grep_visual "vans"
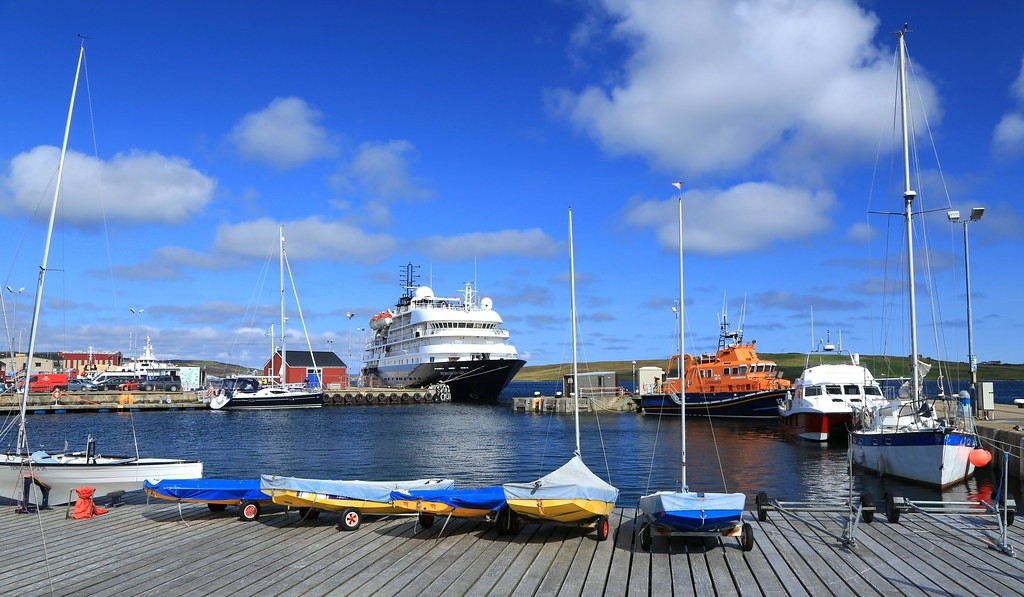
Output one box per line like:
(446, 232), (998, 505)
(16, 373), (68, 394)
(89, 371), (148, 390)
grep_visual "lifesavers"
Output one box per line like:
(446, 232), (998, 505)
(366, 393), (374, 406)
(53, 389), (63, 399)
(424, 393), (432, 404)
(333, 393), (342, 406)
(355, 393), (364, 406)
(413, 393), (421, 405)
(344, 393), (353, 406)
(401, 393), (409, 405)
(390, 392), (398, 406)
(321, 393), (331, 407)
(378, 393), (386, 406)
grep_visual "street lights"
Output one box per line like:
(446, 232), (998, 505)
(947, 207), (986, 417)
(129, 307), (144, 379)
(6, 286), (26, 395)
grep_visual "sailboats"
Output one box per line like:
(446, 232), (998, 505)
(846, 19), (985, 491)
(202, 223), (327, 409)
(639, 194), (746, 532)
(388, 200), (620, 519)
(0, 30), (212, 508)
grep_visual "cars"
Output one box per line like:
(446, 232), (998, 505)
(67, 378), (92, 392)
(98, 374), (182, 394)
(81, 378), (98, 392)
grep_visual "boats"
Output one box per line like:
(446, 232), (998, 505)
(260, 474), (456, 517)
(141, 478), (272, 507)
(775, 303), (890, 442)
(637, 288), (796, 422)
(357, 254), (527, 403)
